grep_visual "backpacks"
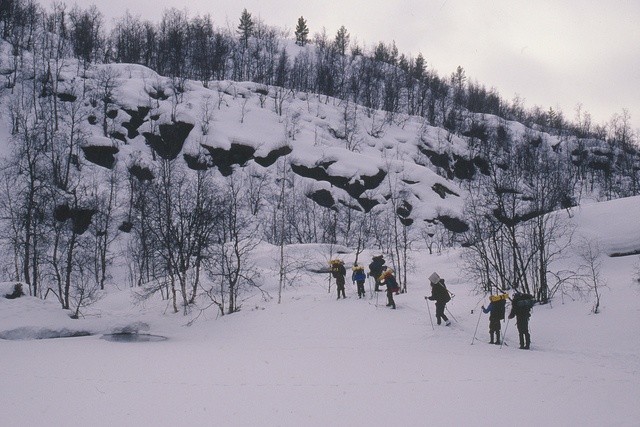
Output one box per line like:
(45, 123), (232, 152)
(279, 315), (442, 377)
(494, 299), (506, 320)
(332, 265), (342, 278)
(515, 299), (531, 311)
(368, 261), (384, 273)
(355, 271), (364, 280)
(435, 286), (450, 303)
(386, 274), (397, 292)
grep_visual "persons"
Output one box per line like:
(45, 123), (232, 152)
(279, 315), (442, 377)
(331, 260), (346, 298)
(481, 294), (509, 345)
(425, 272), (452, 326)
(377, 271), (400, 309)
(352, 265), (366, 298)
(369, 255), (387, 291)
(507, 292), (537, 349)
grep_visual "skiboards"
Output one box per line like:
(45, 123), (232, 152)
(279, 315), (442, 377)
(475, 338), (509, 345)
(368, 302), (404, 309)
